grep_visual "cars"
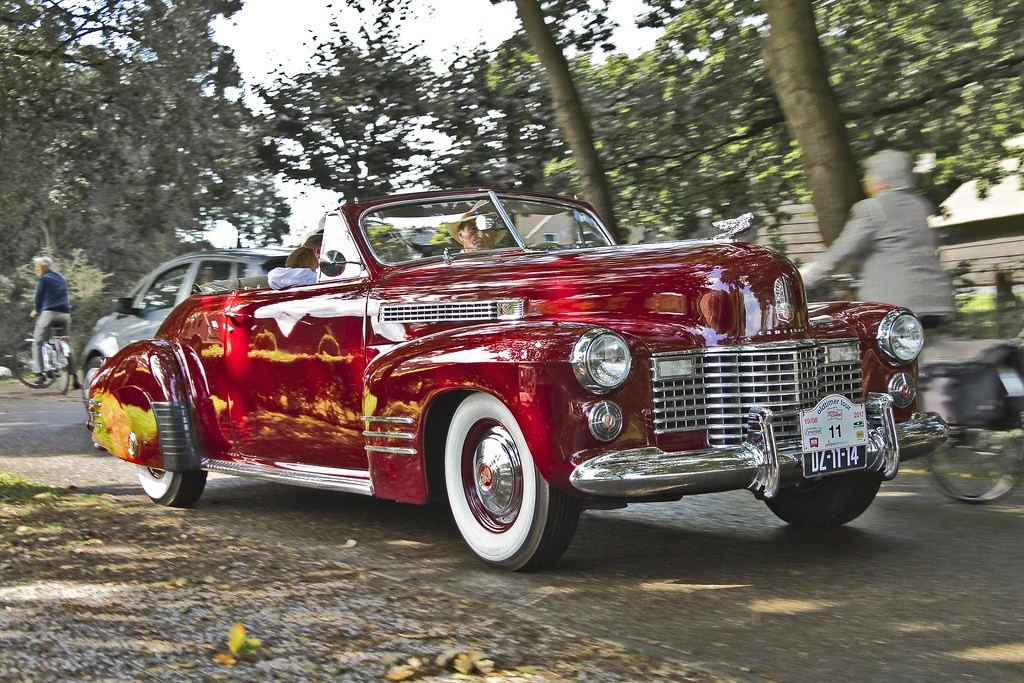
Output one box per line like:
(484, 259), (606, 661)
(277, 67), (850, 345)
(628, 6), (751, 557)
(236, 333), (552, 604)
(86, 187), (949, 576)
(79, 246), (302, 412)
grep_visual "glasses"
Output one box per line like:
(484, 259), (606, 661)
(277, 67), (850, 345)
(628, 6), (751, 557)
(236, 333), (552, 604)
(859, 174), (876, 184)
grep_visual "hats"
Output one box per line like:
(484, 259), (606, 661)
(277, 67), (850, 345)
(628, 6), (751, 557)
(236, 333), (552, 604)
(285, 214), (339, 268)
(444, 200), (508, 246)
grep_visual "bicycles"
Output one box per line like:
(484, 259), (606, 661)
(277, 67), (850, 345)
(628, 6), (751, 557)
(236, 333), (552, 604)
(827, 272), (1024, 504)
(11, 313), (73, 395)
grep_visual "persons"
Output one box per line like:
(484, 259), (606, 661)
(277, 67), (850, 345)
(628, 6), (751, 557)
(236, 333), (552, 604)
(796, 149), (956, 329)
(30, 257), (84, 391)
(268, 214), (325, 290)
(444, 206), (508, 257)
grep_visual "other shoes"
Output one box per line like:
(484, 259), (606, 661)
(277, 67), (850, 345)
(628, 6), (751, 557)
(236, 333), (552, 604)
(34, 375), (46, 384)
(73, 381), (83, 389)
